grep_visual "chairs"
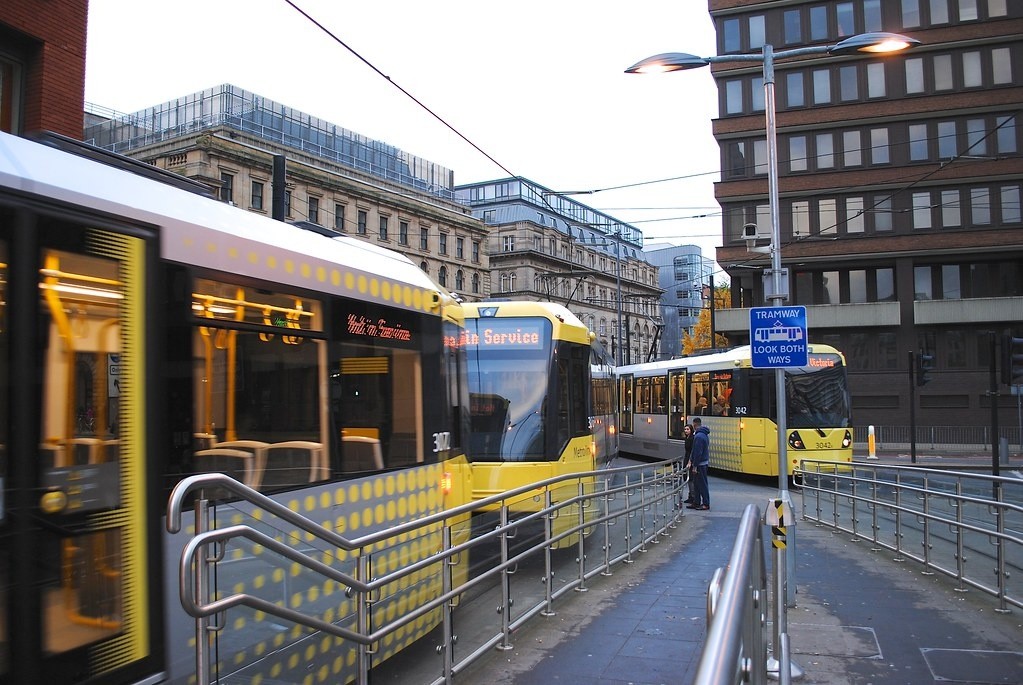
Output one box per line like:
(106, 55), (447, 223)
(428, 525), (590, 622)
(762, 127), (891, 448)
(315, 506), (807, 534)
(38, 431), (388, 629)
(631, 401), (729, 416)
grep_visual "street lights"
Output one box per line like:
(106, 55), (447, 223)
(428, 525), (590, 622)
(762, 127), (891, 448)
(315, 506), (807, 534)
(617, 32), (922, 611)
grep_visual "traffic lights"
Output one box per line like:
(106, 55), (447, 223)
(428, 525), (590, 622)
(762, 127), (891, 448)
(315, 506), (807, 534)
(916, 350), (933, 386)
(1000, 328), (1023, 387)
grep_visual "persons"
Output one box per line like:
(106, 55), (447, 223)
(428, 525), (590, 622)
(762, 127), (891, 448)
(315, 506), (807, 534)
(686, 418), (710, 511)
(656, 392), (731, 417)
(683, 424), (695, 504)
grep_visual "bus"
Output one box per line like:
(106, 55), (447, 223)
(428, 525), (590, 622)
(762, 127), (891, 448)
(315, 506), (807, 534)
(616, 342), (853, 487)
(460, 299), (621, 554)
(0, 127), (474, 685)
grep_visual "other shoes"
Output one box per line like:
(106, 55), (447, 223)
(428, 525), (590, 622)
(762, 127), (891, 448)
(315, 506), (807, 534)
(684, 499), (693, 503)
(695, 504), (709, 510)
(686, 503), (700, 509)
